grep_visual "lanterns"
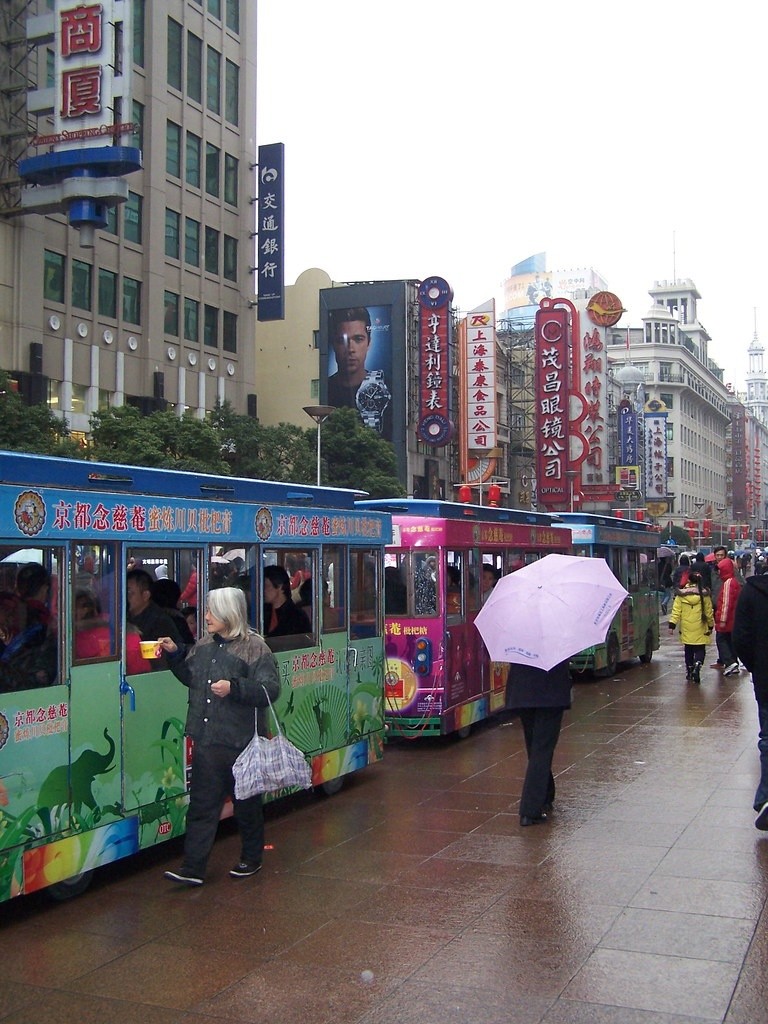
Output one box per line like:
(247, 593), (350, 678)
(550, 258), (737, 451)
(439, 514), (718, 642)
(488, 484), (501, 507)
(460, 486), (472, 504)
(615, 510), (762, 541)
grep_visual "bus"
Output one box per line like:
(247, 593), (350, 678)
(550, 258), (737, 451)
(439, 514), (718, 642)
(1, 451), (392, 909)
(548, 512), (661, 678)
(356, 498), (572, 747)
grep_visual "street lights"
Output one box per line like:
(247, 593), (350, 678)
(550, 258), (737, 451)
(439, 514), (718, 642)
(761, 518), (767, 547)
(736, 511), (742, 545)
(749, 515), (756, 543)
(717, 508), (726, 545)
(302, 406), (336, 486)
(663, 496), (676, 540)
(622, 486), (636, 521)
(694, 502), (704, 551)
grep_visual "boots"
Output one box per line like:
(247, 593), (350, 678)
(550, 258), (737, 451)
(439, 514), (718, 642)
(691, 661), (704, 682)
(686, 666), (694, 681)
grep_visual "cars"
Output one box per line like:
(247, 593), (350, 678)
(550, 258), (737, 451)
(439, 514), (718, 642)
(735, 539), (757, 548)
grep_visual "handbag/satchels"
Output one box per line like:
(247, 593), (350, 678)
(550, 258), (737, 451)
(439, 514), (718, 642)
(232, 684), (313, 799)
(658, 579), (665, 592)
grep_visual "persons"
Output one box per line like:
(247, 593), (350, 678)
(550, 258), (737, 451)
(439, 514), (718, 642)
(385, 566), (407, 615)
(297, 577), (339, 629)
(737, 555), (768, 575)
(504, 661), (575, 827)
(730, 555), (768, 831)
(157, 588), (281, 884)
(483, 563), (499, 607)
(290, 559), (313, 589)
(327, 306), (392, 441)
(127, 569), (184, 672)
(182, 607), (197, 643)
(426, 556), (461, 593)
(0, 562), (59, 694)
(177, 562), (197, 609)
(402, 555), (436, 615)
(73, 587), (153, 675)
(151, 579), (195, 645)
(263, 564), (312, 637)
(658, 546), (747, 683)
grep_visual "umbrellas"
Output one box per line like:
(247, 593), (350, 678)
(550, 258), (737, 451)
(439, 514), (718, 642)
(727, 550), (753, 555)
(657, 547), (675, 557)
(474, 553), (630, 672)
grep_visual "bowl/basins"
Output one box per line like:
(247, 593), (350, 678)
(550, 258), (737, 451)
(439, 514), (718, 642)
(139, 641), (163, 659)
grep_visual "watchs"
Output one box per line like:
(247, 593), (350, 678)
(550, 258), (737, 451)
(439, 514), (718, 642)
(356, 369), (391, 433)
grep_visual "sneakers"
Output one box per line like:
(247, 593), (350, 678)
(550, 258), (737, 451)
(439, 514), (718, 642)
(229, 862), (262, 877)
(163, 867), (204, 886)
(723, 663), (740, 676)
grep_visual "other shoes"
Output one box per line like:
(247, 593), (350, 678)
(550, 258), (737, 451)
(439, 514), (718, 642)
(710, 663), (726, 668)
(542, 802), (553, 813)
(755, 802), (768, 830)
(661, 603), (667, 615)
(521, 814), (547, 826)
